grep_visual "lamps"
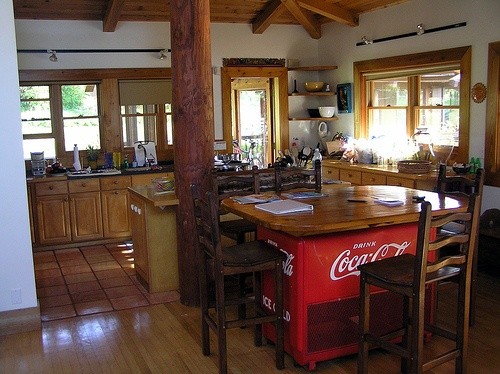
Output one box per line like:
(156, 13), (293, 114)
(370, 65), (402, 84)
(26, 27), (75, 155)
(160, 49), (168, 60)
(417, 24), (424, 35)
(361, 35), (373, 44)
(47, 49), (57, 62)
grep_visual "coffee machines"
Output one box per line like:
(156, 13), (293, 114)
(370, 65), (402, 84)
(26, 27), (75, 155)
(30, 152), (45, 175)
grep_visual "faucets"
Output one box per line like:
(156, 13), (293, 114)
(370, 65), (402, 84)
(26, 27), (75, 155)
(137, 144), (149, 167)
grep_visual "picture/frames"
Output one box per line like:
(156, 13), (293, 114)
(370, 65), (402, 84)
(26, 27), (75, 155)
(336, 83), (353, 113)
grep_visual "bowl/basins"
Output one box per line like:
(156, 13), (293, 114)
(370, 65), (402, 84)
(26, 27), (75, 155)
(317, 106), (337, 118)
(151, 176), (173, 192)
(304, 82), (325, 91)
(306, 109), (320, 118)
(452, 166), (471, 173)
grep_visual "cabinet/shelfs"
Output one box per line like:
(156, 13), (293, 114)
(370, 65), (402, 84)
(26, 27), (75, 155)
(362, 172), (386, 184)
(339, 168), (362, 184)
(322, 166), (340, 180)
(389, 176), (415, 189)
(288, 66), (338, 121)
(26, 172), (179, 293)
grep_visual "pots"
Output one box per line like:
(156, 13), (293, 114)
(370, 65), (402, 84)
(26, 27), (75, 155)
(230, 152), (241, 161)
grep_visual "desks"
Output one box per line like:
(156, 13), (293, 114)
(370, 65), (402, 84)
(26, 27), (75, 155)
(220, 185), (470, 374)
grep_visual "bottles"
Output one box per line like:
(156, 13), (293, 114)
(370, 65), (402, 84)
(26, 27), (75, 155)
(326, 84), (331, 92)
(291, 143), (299, 164)
(104, 149), (124, 167)
(312, 148), (322, 185)
(470, 157), (475, 173)
(474, 158), (481, 174)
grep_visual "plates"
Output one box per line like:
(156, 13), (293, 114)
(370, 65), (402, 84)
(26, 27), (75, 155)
(46, 173), (67, 176)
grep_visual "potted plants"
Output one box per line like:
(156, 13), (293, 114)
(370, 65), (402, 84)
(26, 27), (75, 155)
(86, 145), (100, 169)
(470, 158), (481, 179)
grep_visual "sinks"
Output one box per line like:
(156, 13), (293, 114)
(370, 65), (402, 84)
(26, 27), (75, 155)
(66, 170), (88, 177)
(89, 169), (122, 176)
(125, 167), (151, 172)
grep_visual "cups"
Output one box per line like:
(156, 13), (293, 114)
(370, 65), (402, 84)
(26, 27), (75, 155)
(223, 153), (230, 162)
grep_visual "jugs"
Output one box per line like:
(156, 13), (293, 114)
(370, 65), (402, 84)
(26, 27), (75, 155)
(31, 160), (44, 174)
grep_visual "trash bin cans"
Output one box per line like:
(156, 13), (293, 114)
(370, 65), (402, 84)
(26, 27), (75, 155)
(477, 208), (500, 278)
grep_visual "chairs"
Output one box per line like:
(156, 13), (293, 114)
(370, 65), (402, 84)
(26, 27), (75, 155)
(189, 159), (485, 374)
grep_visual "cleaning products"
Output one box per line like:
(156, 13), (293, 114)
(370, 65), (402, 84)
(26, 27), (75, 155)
(124, 154), (138, 168)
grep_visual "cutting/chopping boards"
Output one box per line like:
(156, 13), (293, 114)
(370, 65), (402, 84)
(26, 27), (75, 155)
(289, 137), (306, 150)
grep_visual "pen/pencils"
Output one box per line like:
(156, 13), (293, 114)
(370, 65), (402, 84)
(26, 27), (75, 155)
(347, 200), (367, 202)
